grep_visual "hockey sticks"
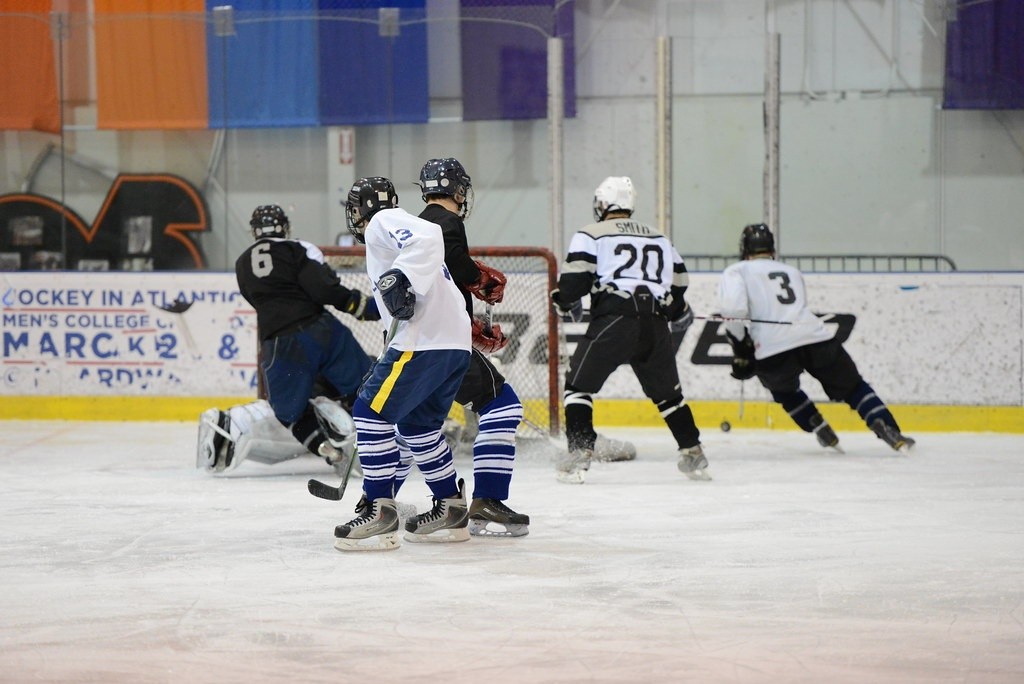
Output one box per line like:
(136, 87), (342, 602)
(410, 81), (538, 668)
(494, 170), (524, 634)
(739, 379), (745, 423)
(307, 317), (400, 501)
(484, 300), (492, 337)
(581, 308), (792, 325)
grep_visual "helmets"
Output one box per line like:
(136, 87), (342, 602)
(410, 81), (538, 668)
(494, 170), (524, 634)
(592, 176), (635, 223)
(739, 222), (774, 260)
(419, 158), (474, 218)
(345, 176), (398, 244)
(250, 205), (289, 240)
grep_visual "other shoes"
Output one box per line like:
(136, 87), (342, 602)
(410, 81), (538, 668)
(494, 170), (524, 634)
(196, 407), (229, 468)
(309, 396), (355, 447)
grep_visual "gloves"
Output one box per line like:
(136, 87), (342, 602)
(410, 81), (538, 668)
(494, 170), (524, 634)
(374, 268), (416, 320)
(726, 326), (756, 380)
(472, 321), (506, 353)
(662, 302), (693, 333)
(465, 260), (507, 306)
(549, 289), (582, 323)
(349, 289), (381, 321)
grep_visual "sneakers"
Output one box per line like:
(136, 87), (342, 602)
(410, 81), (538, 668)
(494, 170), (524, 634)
(334, 482), (401, 551)
(677, 444), (712, 481)
(467, 497), (529, 537)
(555, 448), (593, 484)
(355, 493), (417, 531)
(810, 413), (845, 453)
(868, 418), (915, 457)
(403, 478), (471, 543)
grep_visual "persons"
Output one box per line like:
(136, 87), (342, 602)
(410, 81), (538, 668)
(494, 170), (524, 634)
(202, 395), (461, 476)
(334, 176), (474, 540)
(235, 205), (381, 477)
(721, 223), (916, 455)
(355, 158), (529, 525)
(550, 176), (709, 473)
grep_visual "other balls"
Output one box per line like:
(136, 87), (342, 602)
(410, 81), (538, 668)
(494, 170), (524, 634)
(721, 421), (730, 432)
